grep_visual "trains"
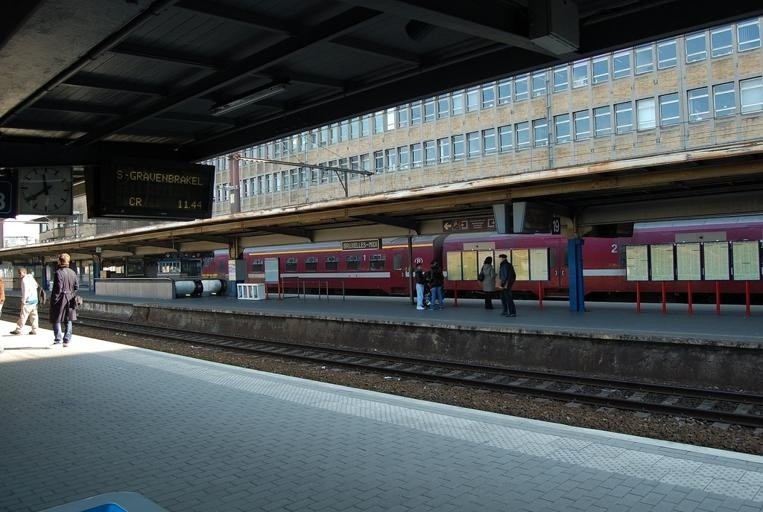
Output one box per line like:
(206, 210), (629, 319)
(199, 215), (763, 298)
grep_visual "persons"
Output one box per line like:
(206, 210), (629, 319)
(415, 263), (425, 309)
(10, 268), (38, 335)
(482, 257), (498, 309)
(49, 253), (79, 347)
(499, 255), (516, 317)
(426, 260), (444, 310)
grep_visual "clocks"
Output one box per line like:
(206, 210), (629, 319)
(20, 165), (73, 217)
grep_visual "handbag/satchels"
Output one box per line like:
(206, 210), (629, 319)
(72, 295), (83, 308)
(478, 273), (484, 281)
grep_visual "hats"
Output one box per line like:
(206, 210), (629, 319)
(499, 254), (506, 257)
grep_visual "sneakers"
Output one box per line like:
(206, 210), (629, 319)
(505, 314), (515, 317)
(29, 332), (35, 334)
(10, 331), (18, 334)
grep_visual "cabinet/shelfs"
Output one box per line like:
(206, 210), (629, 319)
(236, 282), (267, 301)
